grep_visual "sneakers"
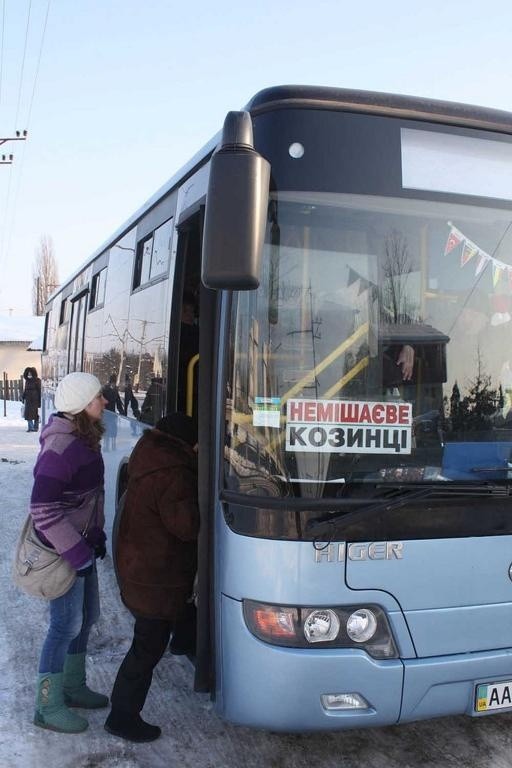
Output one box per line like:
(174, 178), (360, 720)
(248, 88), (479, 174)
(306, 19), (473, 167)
(102, 708), (161, 741)
(170, 632), (196, 654)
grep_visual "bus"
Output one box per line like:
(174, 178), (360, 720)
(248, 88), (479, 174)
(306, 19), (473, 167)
(36, 82), (512, 735)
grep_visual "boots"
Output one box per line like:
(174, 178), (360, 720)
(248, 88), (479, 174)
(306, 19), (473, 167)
(33, 672), (89, 733)
(62, 652), (108, 707)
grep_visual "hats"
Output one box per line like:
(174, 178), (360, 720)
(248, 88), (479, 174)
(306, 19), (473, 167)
(55, 373), (102, 415)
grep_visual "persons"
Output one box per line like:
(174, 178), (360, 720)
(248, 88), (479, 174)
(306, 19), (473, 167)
(20, 367), (41, 432)
(104, 413), (199, 742)
(180, 289), (198, 375)
(30, 370), (109, 734)
(396, 343), (417, 381)
(101, 374), (166, 451)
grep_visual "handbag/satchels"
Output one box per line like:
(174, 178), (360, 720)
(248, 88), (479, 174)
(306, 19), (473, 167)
(12, 514), (88, 600)
